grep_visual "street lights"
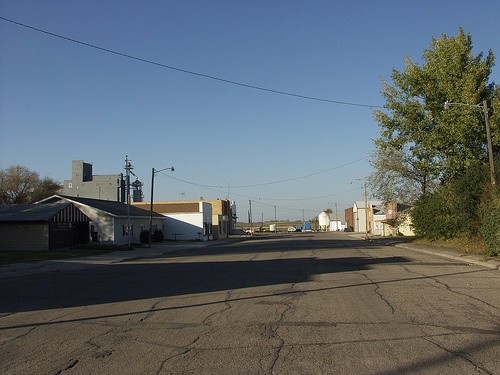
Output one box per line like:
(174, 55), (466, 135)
(444, 98), (494, 184)
(349, 181), (368, 239)
(148, 167), (176, 248)
